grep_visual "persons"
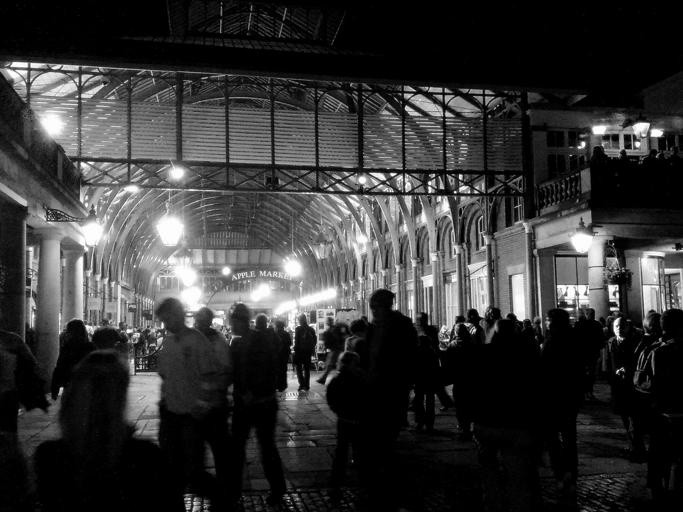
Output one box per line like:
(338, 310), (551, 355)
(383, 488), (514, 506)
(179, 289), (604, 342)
(584, 144), (683, 168)
(0, 288), (683, 512)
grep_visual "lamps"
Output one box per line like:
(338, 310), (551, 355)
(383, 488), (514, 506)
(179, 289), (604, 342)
(311, 194), (333, 260)
(620, 113), (650, 137)
(43, 205), (102, 247)
(156, 180), (202, 309)
